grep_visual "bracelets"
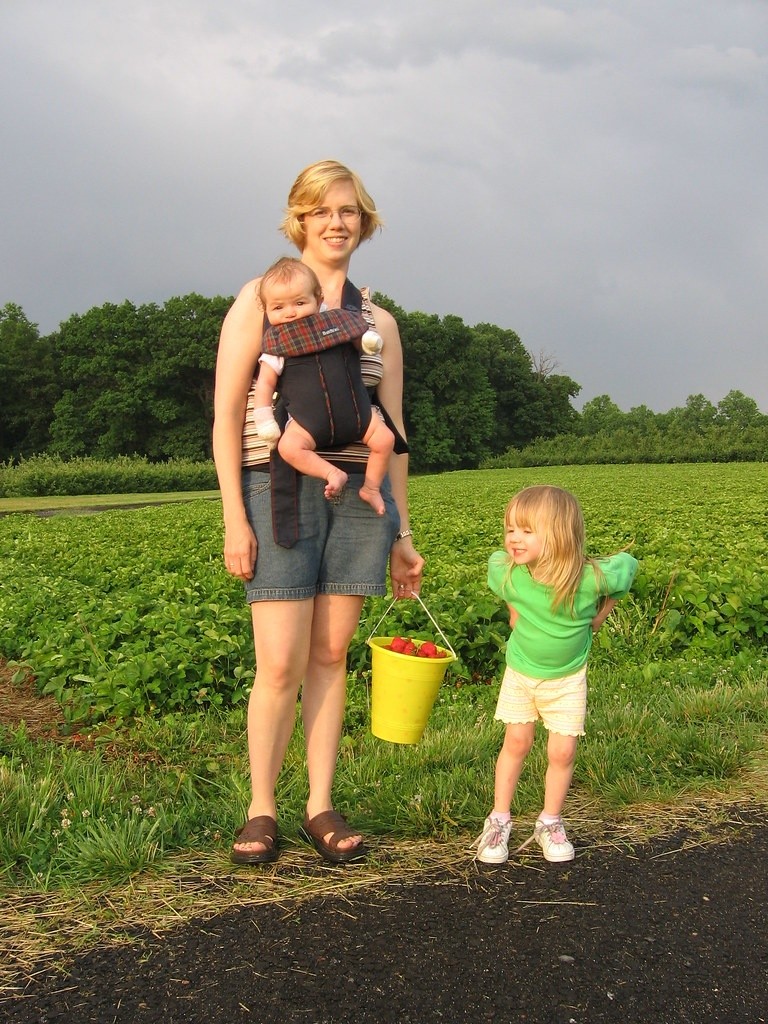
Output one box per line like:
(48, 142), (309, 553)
(396, 530), (413, 542)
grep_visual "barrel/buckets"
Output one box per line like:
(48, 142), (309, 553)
(364, 590), (457, 746)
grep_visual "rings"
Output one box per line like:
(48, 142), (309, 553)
(230, 564), (234, 566)
(399, 585), (404, 588)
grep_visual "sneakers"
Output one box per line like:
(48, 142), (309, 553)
(530, 819), (574, 863)
(468, 812), (513, 866)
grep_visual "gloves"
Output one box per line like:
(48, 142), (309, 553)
(363, 330), (384, 356)
(251, 406), (282, 449)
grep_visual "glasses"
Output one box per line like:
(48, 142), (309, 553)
(308, 205), (362, 224)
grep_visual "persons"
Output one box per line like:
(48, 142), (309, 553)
(474, 486), (637, 863)
(210, 161), (424, 864)
(251, 257), (395, 514)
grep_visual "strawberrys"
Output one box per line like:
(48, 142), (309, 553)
(379, 635), (447, 658)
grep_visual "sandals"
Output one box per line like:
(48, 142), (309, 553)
(298, 805), (366, 863)
(232, 815), (280, 864)
